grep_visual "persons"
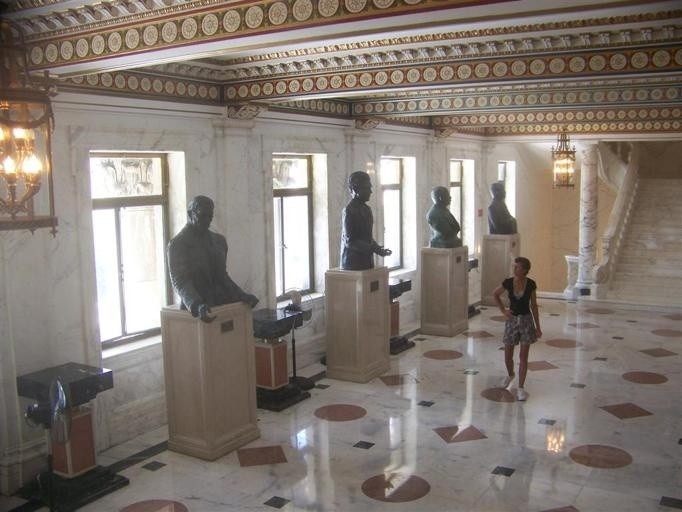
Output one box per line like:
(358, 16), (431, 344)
(491, 256), (542, 401)
(486, 182), (517, 234)
(425, 185), (462, 247)
(340, 170), (392, 270)
(167, 194), (259, 323)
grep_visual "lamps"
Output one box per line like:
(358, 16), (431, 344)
(0, 15), (61, 239)
(550, 122), (576, 190)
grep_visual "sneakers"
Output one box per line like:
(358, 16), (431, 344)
(517, 388), (526, 400)
(501, 372), (516, 389)
